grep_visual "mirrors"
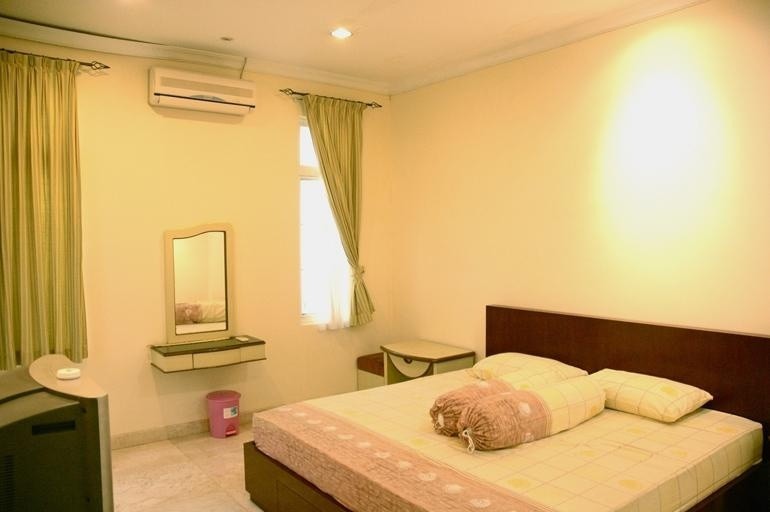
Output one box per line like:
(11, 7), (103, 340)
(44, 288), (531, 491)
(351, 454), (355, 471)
(165, 223), (232, 342)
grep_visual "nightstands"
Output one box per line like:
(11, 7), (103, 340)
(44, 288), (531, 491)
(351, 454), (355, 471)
(380, 339), (475, 385)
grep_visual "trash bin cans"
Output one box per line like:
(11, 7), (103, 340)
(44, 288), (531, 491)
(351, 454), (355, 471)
(205, 390), (241, 438)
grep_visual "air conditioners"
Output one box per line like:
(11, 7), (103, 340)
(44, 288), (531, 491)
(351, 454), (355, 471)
(146, 65), (256, 115)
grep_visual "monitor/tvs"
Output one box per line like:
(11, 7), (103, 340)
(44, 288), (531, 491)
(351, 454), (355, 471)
(0, 353), (114, 512)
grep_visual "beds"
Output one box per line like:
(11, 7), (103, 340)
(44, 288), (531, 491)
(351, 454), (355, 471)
(244, 304), (769, 512)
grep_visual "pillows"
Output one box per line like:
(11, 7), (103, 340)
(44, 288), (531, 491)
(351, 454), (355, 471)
(429, 352), (713, 454)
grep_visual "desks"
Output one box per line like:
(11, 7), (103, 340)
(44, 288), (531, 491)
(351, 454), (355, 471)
(149, 334), (266, 374)
